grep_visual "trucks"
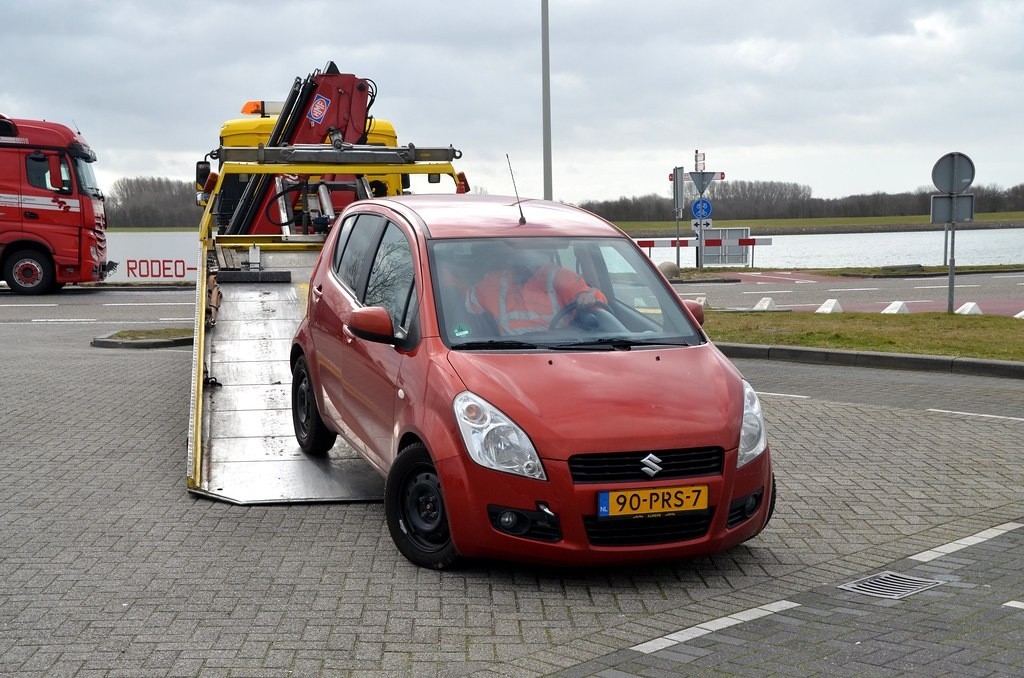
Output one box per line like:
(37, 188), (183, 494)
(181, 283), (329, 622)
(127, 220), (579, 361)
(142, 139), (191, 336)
(0, 112), (119, 294)
(185, 57), (476, 506)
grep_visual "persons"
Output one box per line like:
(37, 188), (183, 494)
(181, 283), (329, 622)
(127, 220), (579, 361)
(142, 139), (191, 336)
(440, 242), (607, 338)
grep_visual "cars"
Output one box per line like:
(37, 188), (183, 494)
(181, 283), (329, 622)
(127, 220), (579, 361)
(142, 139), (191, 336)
(288, 193), (778, 572)
